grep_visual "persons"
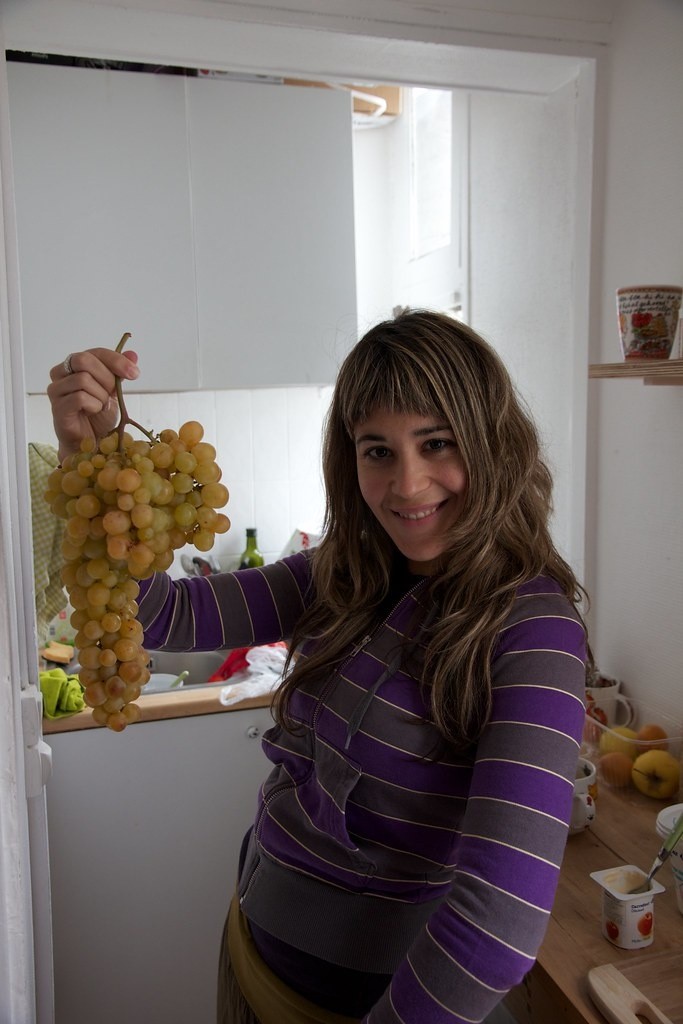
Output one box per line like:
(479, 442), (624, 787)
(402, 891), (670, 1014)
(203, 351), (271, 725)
(47, 312), (594, 1024)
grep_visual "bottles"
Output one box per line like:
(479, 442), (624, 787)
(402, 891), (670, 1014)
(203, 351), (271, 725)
(238, 528), (264, 571)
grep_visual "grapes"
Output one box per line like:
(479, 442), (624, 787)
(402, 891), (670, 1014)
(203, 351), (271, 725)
(43, 333), (233, 733)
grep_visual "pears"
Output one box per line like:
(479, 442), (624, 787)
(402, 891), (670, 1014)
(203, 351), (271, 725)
(631, 751), (681, 797)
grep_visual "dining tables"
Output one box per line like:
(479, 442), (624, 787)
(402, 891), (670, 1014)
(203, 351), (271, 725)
(503, 741), (683, 1024)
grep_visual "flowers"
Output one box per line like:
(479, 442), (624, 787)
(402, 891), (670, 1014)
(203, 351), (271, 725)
(630, 312), (657, 353)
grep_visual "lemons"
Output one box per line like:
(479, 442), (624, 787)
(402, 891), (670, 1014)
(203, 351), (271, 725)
(600, 727), (637, 757)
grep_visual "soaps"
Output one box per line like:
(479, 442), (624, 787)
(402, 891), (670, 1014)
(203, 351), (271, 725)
(42, 638), (75, 665)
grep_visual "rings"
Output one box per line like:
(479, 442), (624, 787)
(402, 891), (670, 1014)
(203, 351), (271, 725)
(64, 353), (75, 374)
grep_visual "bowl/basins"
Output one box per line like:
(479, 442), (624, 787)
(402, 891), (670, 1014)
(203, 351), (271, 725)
(139, 673), (184, 692)
(581, 696), (683, 808)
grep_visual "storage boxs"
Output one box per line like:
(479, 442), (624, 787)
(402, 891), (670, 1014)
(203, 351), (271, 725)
(579, 696), (683, 805)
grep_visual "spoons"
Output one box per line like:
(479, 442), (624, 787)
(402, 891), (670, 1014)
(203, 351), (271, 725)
(625, 810), (683, 896)
(180, 553), (222, 578)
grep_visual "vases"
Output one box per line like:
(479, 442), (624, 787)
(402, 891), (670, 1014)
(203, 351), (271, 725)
(618, 285), (683, 363)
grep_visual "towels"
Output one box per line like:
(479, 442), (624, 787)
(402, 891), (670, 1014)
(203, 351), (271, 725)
(36, 667), (94, 719)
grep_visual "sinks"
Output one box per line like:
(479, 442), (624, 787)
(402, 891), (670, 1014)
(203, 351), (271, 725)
(46, 647), (253, 697)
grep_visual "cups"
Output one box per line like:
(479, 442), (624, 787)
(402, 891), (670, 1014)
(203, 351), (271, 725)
(614, 284), (683, 362)
(583, 671), (636, 740)
(566, 756), (597, 835)
(654, 802), (682, 917)
